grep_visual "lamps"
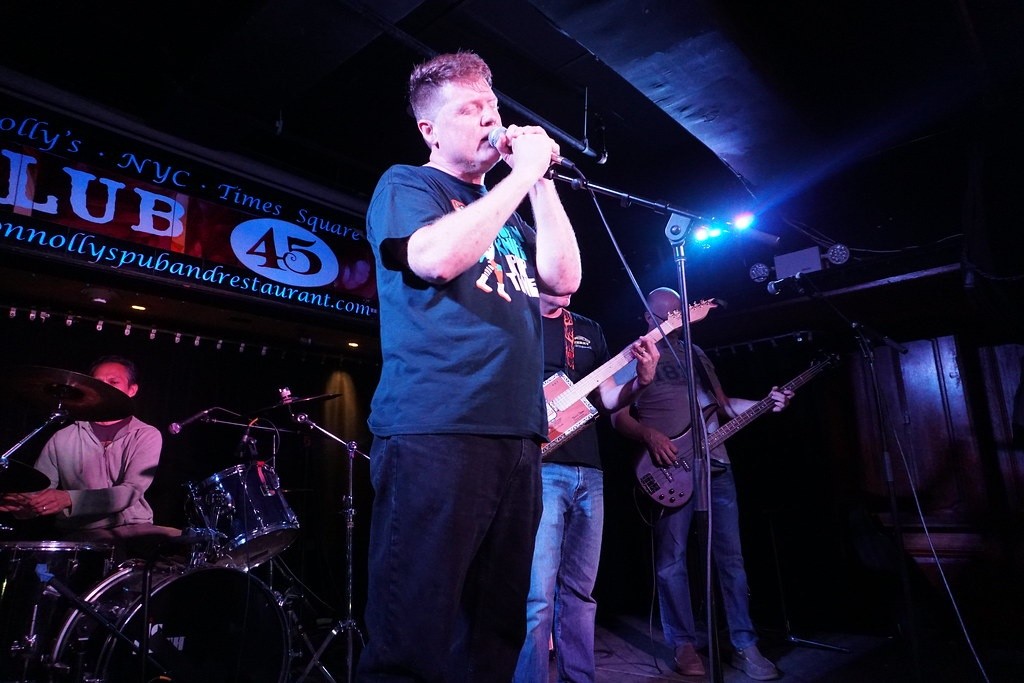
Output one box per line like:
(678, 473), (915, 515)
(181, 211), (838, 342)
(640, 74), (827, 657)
(749, 244), (850, 283)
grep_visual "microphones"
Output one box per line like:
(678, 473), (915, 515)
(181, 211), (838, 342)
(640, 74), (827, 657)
(488, 126), (575, 168)
(169, 408), (213, 434)
(237, 428), (252, 458)
(768, 272), (803, 294)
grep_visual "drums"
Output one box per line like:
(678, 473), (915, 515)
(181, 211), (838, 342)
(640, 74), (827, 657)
(48, 559), (292, 683)
(0, 540), (120, 683)
(183, 462), (301, 573)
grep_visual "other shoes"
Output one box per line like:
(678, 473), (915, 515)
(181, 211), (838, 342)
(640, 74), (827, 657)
(732, 645), (778, 679)
(674, 643), (704, 675)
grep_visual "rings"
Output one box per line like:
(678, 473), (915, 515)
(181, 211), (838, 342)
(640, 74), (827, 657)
(44, 507), (46, 509)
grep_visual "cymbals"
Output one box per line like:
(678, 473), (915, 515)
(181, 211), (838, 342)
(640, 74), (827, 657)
(0, 364), (136, 421)
(240, 392), (344, 418)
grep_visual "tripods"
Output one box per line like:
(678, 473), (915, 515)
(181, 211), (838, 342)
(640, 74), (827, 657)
(286, 402), (373, 683)
(754, 504), (854, 661)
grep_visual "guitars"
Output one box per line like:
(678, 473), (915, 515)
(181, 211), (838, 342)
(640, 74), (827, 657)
(635, 348), (843, 511)
(540, 296), (717, 460)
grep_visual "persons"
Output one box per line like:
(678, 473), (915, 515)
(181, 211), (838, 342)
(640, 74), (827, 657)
(365, 54), (585, 682)
(1, 358), (162, 537)
(515, 293), (660, 680)
(609, 289), (798, 679)
